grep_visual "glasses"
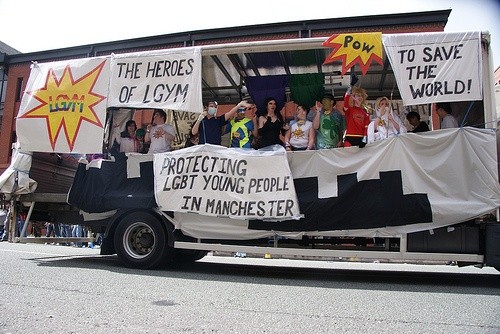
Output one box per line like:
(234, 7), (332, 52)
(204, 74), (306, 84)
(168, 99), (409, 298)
(154, 114), (160, 118)
(244, 107), (254, 111)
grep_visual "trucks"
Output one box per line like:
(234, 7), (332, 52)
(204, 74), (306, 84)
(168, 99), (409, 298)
(1, 28), (500, 270)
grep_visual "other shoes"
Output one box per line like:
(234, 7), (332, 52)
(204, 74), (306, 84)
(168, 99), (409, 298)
(0, 238), (8, 242)
(45, 241), (94, 249)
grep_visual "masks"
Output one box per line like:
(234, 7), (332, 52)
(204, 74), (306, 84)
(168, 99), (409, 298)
(207, 108), (217, 115)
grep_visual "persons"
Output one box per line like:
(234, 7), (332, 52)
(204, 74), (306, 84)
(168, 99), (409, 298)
(103, 87), (464, 155)
(0, 196), (104, 248)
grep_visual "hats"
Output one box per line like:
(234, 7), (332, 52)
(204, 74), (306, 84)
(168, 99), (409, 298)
(320, 93), (336, 106)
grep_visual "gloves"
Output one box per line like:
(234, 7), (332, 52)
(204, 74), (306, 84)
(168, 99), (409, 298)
(197, 113), (205, 121)
(237, 100), (249, 108)
